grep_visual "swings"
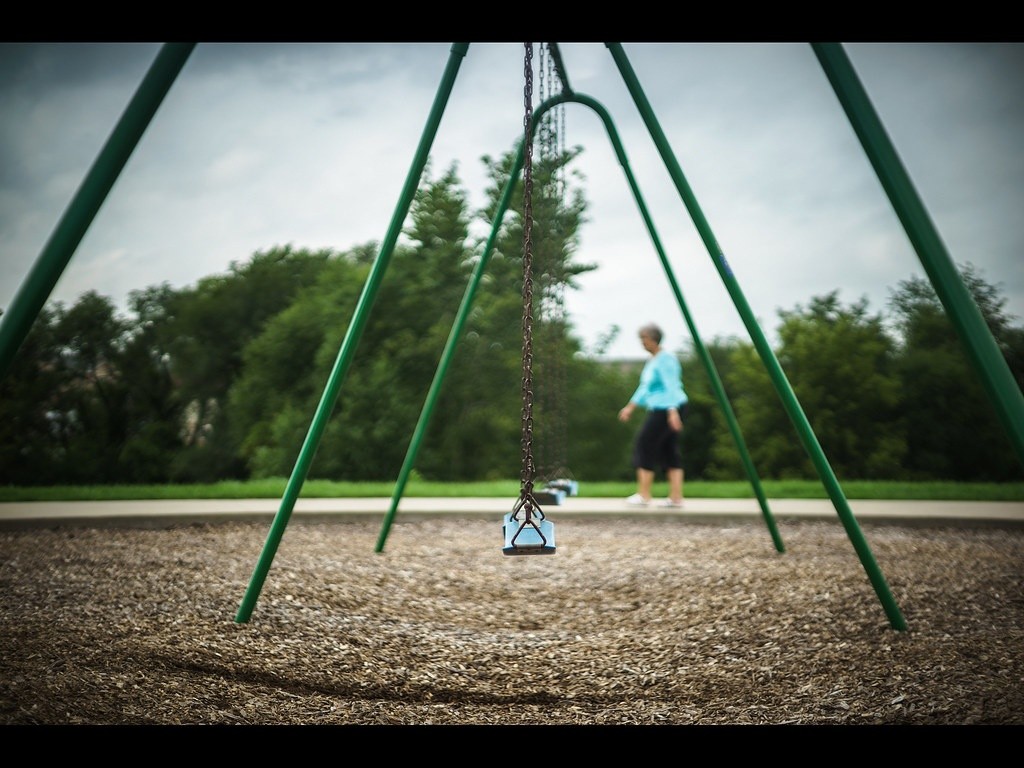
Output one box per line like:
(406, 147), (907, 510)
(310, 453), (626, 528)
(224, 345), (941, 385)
(501, 43), (586, 560)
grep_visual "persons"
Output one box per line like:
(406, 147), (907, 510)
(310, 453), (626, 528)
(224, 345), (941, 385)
(618, 324), (688, 508)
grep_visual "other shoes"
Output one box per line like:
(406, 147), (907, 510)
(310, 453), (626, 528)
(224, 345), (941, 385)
(660, 497), (683, 509)
(625, 493), (652, 508)
